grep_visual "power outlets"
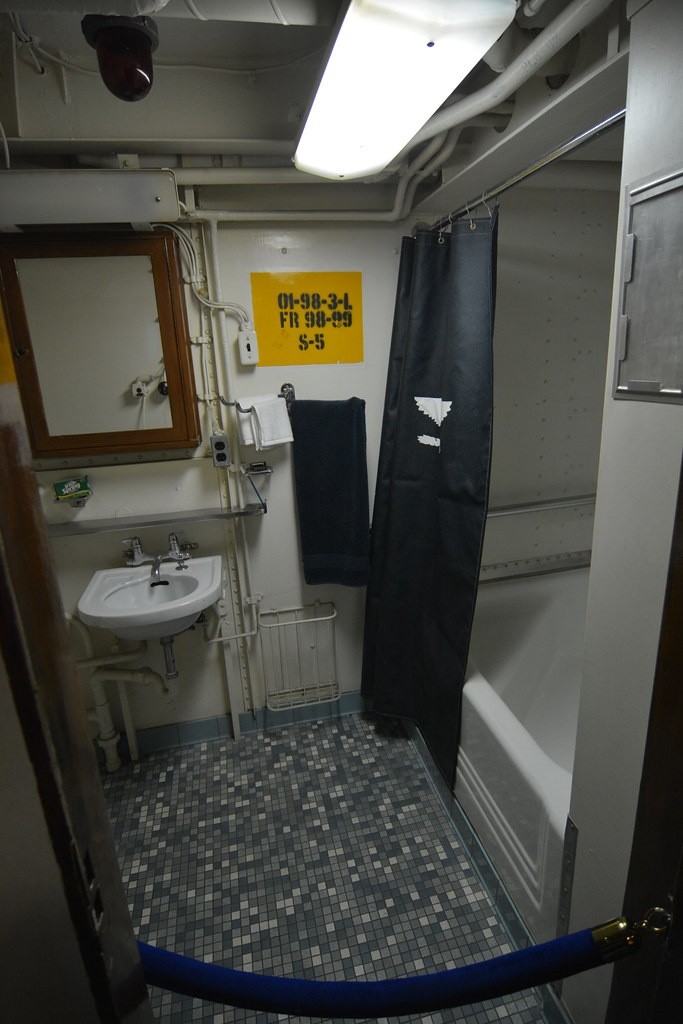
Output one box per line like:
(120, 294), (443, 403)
(211, 434), (232, 468)
(131, 382), (146, 397)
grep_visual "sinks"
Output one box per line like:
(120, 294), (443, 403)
(76, 554), (223, 641)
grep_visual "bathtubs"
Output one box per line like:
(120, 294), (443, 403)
(457, 564), (594, 872)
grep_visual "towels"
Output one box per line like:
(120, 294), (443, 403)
(234, 393), (295, 453)
(288, 396), (372, 589)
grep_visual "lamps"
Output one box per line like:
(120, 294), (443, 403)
(291, 0), (521, 180)
(81, 13), (159, 101)
(0, 167), (180, 226)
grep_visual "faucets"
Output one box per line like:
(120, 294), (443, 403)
(148, 551), (162, 583)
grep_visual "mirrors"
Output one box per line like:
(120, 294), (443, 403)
(0, 237), (190, 451)
